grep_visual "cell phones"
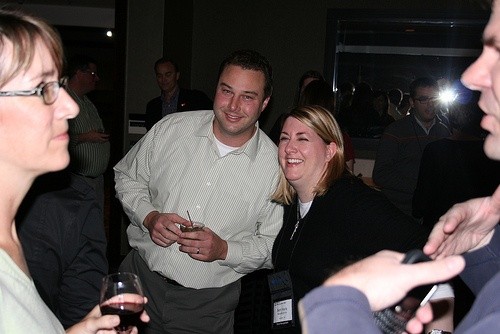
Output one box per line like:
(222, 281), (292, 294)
(371, 249), (438, 334)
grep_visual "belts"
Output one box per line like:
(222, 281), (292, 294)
(153, 271), (184, 287)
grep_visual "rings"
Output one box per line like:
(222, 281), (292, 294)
(196, 248), (200, 254)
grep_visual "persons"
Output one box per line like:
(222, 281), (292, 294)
(266, 105), (429, 334)
(0, 10), (151, 334)
(297, 0), (499, 334)
(270, 71), (355, 175)
(113, 50), (284, 334)
(339, 80), (452, 134)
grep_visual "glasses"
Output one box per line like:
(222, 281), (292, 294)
(0, 76), (71, 105)
(415, 96), (439, 104)
(85, 70), (95, 76)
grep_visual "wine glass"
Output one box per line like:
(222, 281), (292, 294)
(100, 271), (144, 334)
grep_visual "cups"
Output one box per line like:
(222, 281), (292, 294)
(96, 127), (108, 144)
(179, 221), (205, 249)
